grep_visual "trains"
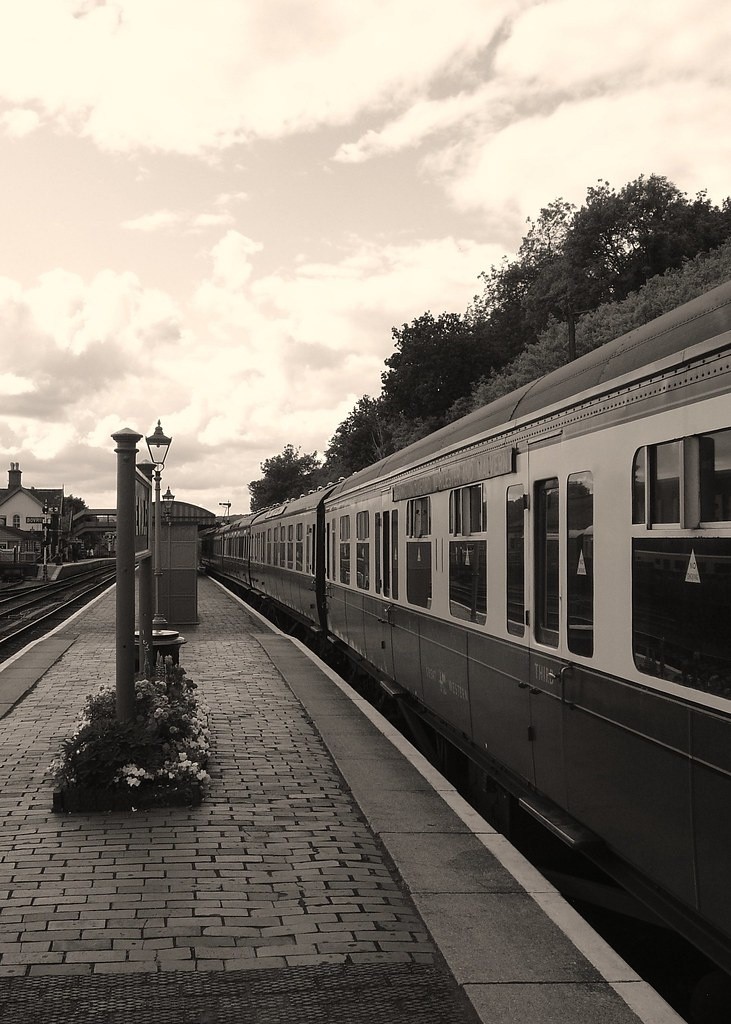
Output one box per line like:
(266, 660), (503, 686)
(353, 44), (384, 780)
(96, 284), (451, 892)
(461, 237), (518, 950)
(199, 281), (731, 975)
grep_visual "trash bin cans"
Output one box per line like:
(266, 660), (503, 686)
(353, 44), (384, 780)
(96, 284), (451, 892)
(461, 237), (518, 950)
(135, 630), (187, 672)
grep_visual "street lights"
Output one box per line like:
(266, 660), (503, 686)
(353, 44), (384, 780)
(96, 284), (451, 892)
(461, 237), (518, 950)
(162, 486), (175, 568)
(145, 419), (172, 612)
